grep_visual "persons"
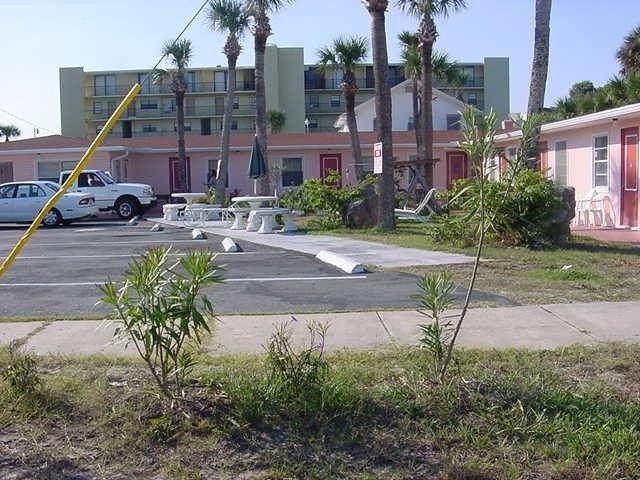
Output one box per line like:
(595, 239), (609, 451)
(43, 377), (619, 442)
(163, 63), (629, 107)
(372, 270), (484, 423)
(206, 172), (213, 189)
(206, 169), (216, 197)
(87, 174), (101, 186)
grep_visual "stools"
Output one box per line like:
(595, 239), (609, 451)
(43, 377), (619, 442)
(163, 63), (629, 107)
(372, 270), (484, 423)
(228, 207), (299, 234)
(162, 203), (221, 222)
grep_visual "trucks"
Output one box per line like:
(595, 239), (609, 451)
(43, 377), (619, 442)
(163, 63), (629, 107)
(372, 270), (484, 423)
(59, 169), (158, 220)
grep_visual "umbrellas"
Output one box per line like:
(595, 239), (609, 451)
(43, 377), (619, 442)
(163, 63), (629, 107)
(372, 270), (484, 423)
(247, 135), (267, 195)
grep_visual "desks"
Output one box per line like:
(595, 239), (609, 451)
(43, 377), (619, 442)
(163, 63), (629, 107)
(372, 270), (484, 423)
(231, 196), (280, 232)
(171, 192), (206, 221)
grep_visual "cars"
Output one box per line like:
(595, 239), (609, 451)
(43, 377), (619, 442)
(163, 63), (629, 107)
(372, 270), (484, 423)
(0, 180), (98, 229)
